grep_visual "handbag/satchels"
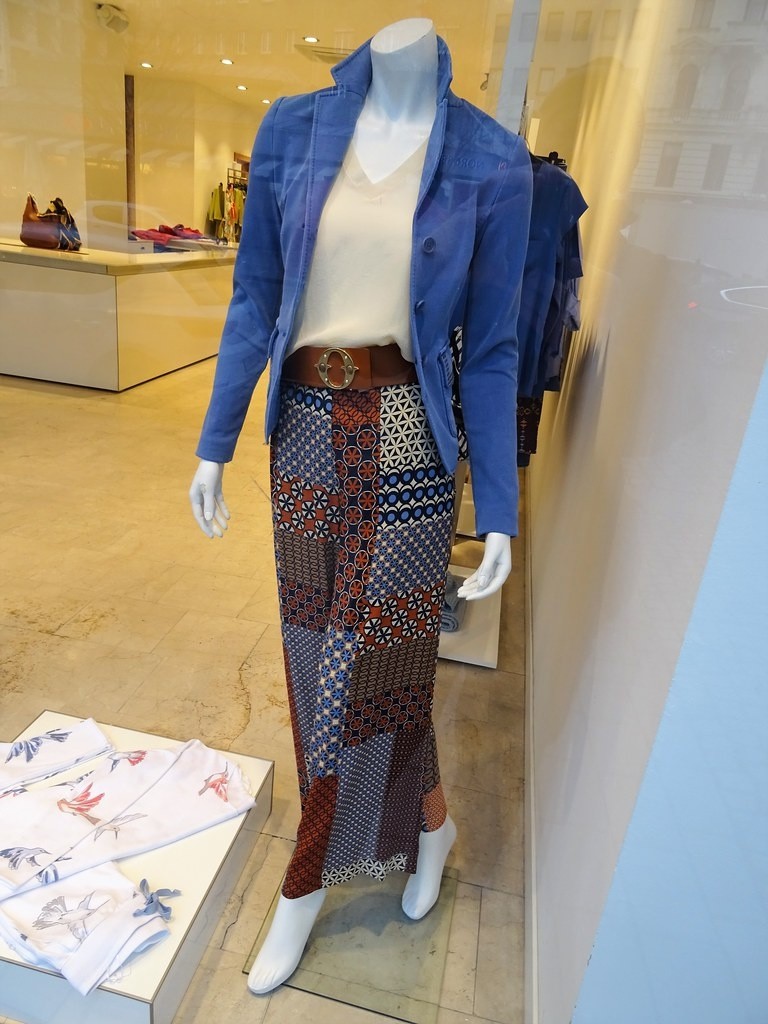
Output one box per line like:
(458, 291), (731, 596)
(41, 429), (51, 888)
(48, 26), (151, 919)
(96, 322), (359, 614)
(20, 192), (82, 252)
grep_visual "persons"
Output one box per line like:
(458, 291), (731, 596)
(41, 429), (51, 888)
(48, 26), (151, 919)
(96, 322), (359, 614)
(189, 16), (535, 995)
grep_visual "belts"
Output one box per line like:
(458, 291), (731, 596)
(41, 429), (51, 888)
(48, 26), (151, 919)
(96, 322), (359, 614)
(280, 344), (419, 389)
